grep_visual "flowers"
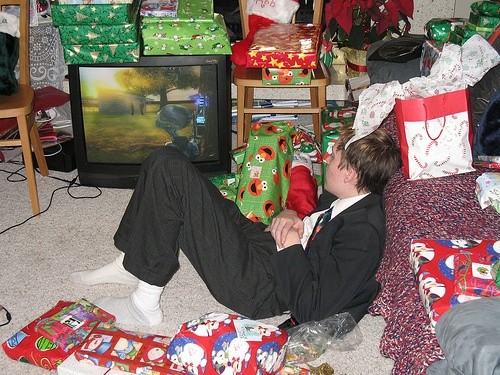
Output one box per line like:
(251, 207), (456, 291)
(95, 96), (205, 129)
(325, 0), (415, 51)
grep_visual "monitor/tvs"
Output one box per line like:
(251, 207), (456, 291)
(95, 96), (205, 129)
(68, 55), (233, 188)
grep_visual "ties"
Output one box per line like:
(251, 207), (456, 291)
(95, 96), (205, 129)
(305, 206), (339, 250)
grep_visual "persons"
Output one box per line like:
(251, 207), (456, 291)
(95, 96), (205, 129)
(70, 124), (402, 326)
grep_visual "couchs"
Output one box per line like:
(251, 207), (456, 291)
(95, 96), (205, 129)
(367, 112), (500, 375)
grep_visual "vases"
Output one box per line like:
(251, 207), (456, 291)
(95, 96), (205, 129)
(344, 45), (367, 77)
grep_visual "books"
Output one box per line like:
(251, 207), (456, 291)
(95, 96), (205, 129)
(251, 114), (299, 123)
(253, 100), (299, 108)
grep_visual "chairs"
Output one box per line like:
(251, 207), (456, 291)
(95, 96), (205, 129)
(234, 0), (340, 183)
(0, 0), (49, 217)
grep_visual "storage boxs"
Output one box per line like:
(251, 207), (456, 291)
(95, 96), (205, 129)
(424, 0), (500, 47)
(50, 0), (232, 64)
(2, 299), (289, 375)
(408, 239), (500, 334)
(246, 23), (322, 84)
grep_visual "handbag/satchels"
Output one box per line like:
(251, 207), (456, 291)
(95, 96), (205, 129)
(394, 84), (477, 182)
